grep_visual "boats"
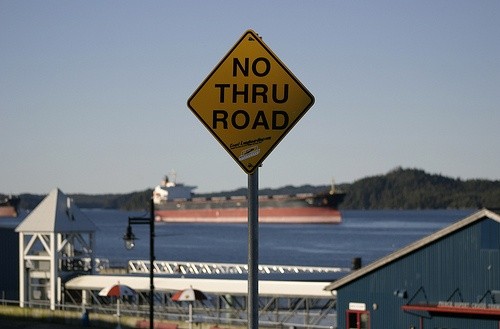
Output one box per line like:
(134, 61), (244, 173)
(151, 175), (347, 225)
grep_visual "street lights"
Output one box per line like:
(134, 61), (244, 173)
(123, 197), (155, 328)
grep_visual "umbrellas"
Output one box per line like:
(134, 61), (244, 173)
(170, 284), (210, 329)
(97, 280), (138, 329)
